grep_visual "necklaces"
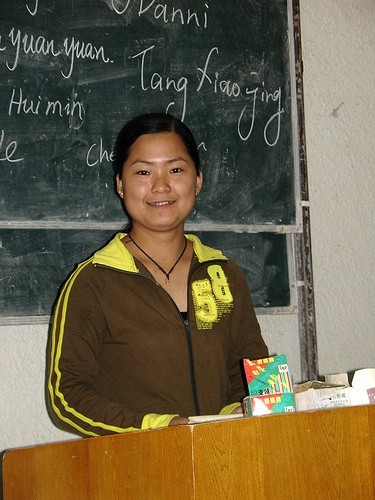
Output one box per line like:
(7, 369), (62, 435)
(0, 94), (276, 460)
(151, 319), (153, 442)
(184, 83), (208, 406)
(128, 232), (188, 285)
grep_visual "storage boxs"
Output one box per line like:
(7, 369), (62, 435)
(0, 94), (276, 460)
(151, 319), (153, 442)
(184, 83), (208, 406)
(243, 357), (375, 416)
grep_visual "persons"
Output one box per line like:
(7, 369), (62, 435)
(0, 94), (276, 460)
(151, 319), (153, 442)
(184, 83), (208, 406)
(44, 111), (269, 438)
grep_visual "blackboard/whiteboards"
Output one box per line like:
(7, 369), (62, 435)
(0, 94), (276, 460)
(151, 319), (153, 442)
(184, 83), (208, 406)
(0, 1), (304, 324)
